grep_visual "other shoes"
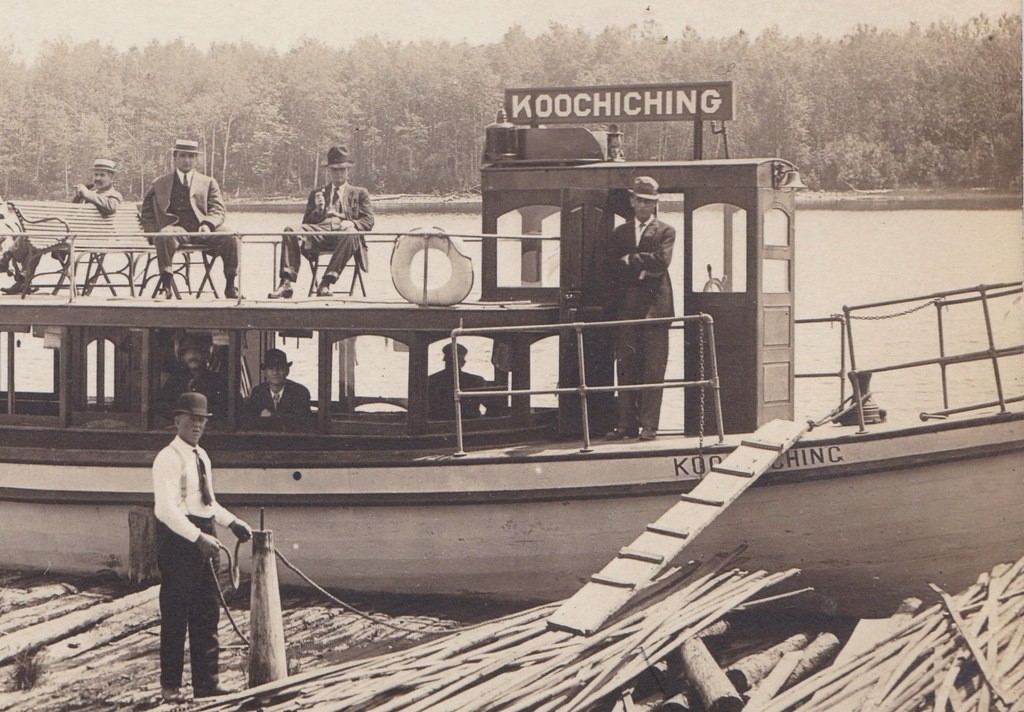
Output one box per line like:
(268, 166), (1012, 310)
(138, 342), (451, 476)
(0, 281), (26, 293)
(0, 258), (9, 272)
(161, 689), (193, 703)
(640, 426), (657, 440)
(193, 684), (241, 698)
(154, 288), (172, 301)
(316, 283), (333, 297)
(605, 426), (638, 440)
(269, 280), (293, 298)
(225, 287), (246, 299)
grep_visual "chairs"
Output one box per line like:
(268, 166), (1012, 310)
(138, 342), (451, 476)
(295, 243), (372, 297)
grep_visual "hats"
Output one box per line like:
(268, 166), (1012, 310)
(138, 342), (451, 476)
(261, 349), (293, 369)
(627, 176), (661, 200)
(171, 139), (203, 153)
(176, 334), (212, 357)
(91, 159), (120, 173)
(174, 393), (212, 416)
(443, 342), (467, 365)
(322, 147), (353, 168)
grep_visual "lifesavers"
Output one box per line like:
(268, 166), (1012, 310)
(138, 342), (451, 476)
(390, 226), (474, 306)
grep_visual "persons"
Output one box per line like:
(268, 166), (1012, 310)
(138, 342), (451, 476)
(141, 138), (247, 299)
(605, 177), (675, 438)
(239, 348), (310, 432)
(268, 145), (375, 299)
(0, 157), (123, 295)
(152, 392), (253, 702)
(429, 343), (494, 420)
(160, 336), (227, 412)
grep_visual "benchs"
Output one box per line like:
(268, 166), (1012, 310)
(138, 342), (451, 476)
(0, 201), (226, 303)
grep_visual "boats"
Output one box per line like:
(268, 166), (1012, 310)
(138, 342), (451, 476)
(1, 74), (1024, 648)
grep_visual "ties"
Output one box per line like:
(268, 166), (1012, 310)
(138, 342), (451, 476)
(273, 394), (279, 411)
(183, 175), (187, 186)
(639, 223), (645, 231)
(333, 187), (343, 214)
(193, 448), (213, 506)
